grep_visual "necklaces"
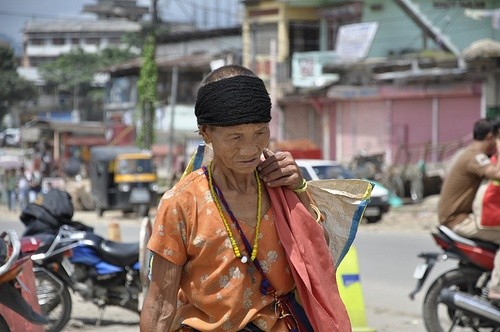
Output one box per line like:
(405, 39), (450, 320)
(207, 158), (263, 267)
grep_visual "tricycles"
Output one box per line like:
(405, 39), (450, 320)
(89, 145), (156, 219)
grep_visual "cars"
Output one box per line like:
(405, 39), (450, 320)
(295, 158), (390, 224)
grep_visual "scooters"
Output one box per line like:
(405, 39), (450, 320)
(0, 224), (96, 332)
(17, 182), (141, 328)
(409, 225), (500, 332)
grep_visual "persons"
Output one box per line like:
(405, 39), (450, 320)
(140, 65), (330, 332)
(438, 118), (500, 300)
(0, 149), (51, 213)
